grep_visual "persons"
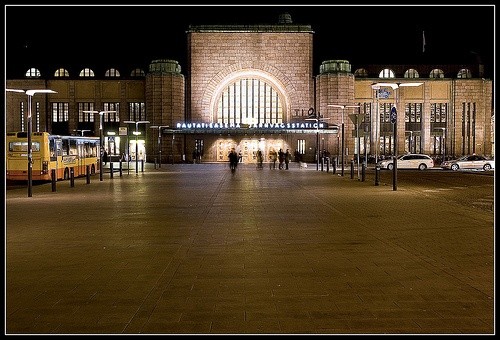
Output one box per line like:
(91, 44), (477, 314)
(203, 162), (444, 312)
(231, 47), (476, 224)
(268, 147), (278, 169)
(284, 149), (293, 170)
(119, 151), (128, 162)
(227, 148), (239, 181)
(278, 148), (285, 171)
(256, 148), (263, 170)
(238, 150), (243, 162)
(192, 149), (197, 164)
(103, 150), (107, 166)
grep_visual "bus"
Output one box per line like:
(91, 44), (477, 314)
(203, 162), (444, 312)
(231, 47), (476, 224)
(6, 132), (99, 182)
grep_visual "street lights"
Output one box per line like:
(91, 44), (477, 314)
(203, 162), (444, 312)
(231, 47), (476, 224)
(328, 105), (362, 176)
(73, 130), (91, 136)
(370, 82), (423, 190)
(305, 118), (330, 171)
(149, 125), (169, 164)
(82, 111), (116, 181)
(123, 121), (150, 173)
(6, 89), (58, 197)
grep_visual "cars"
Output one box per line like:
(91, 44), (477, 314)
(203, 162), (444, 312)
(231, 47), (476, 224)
(440, 155), (495, 171)
(378, 154), (434, 170)
